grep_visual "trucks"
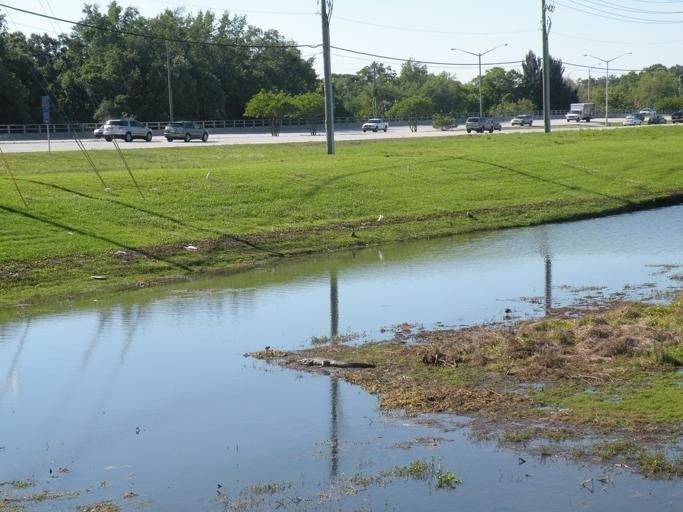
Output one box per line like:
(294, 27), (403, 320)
(565, 102), (596, 122)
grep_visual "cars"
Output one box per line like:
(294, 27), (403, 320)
(465, 116), (502, 133)
(164, 119), (210, 142)
(361, 118), (388, 132)
(623, 107), (683, 126)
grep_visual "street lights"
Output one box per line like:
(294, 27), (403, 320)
(450, 43), (508, 117)
(583, 51), (632, 125)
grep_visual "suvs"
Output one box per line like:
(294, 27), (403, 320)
(93, 119), (153, 142)
(510, 114), (532, 126)
